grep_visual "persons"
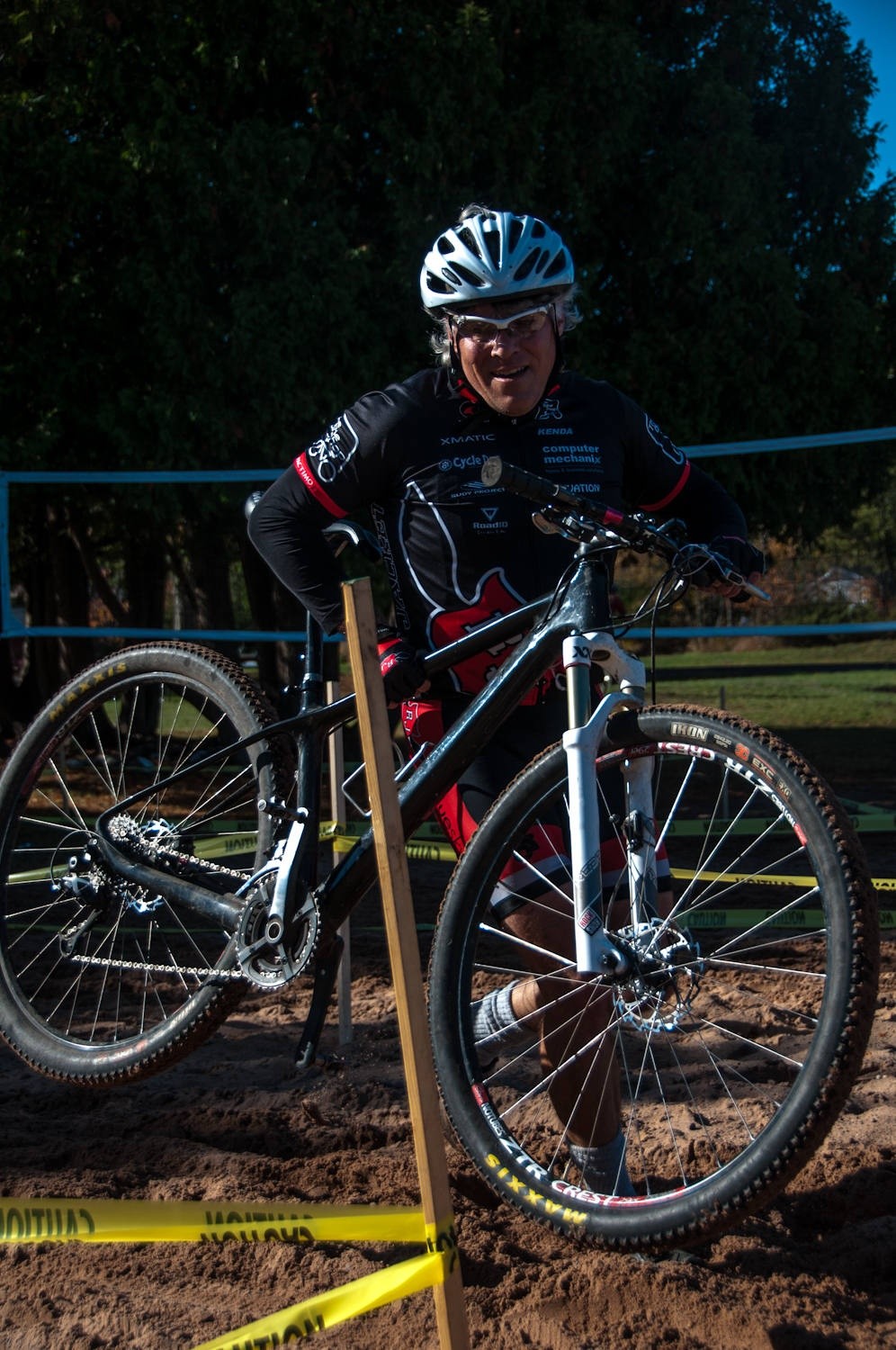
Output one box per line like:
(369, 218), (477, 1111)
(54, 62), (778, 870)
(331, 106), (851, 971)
(247, 206), (764, 1201)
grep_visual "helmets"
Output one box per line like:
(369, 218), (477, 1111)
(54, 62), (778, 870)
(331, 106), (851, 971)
(421, 211), (574, 307)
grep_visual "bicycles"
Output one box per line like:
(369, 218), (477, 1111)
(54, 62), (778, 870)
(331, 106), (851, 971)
(0, 457), (882, 1261)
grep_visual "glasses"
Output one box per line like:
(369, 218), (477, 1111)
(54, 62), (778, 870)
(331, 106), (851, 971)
(441, 295), (552, 343)
